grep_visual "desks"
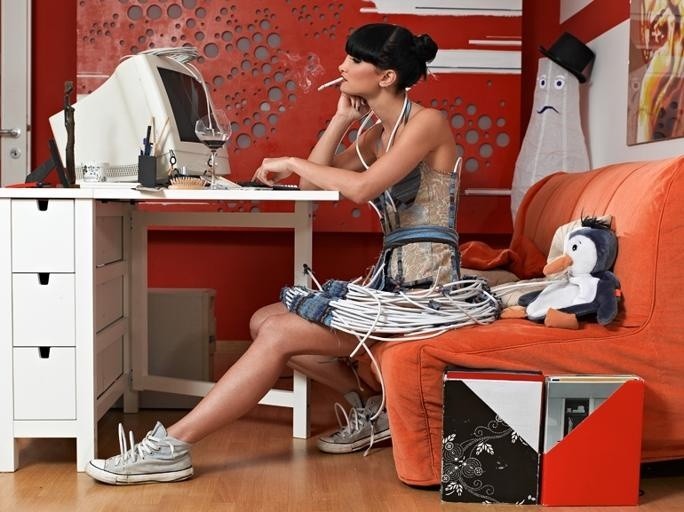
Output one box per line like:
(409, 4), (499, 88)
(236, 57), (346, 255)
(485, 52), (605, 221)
(0, 172), (345, 473)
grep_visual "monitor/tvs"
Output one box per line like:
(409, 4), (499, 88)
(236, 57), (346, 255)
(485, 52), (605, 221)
(48, 54), (231, 185)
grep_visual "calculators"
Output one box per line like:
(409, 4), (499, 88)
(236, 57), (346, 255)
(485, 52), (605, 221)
(236, 182), (299, 190)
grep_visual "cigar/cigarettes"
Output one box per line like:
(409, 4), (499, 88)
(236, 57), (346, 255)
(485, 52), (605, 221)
(317, 77), (343, 91)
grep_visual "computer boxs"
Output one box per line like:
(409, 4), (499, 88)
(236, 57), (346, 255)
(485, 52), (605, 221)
(108, 288), (217, 409)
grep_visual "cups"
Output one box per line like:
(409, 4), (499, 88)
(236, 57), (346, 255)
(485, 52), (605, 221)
(81, 159), (110, 182)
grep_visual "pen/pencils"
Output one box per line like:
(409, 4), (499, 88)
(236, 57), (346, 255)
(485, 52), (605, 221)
(140, 116), (170, 157)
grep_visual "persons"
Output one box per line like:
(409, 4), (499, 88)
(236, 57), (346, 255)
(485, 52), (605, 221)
(84, 24), (460, 486)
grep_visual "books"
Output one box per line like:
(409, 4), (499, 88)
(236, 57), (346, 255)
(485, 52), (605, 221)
(446, 374), (640, 454)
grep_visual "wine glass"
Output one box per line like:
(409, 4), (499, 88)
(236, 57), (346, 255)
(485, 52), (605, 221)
(195, 108), (232, 189)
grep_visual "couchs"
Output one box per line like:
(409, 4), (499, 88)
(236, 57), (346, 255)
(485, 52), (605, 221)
(357, 155), (684, 485)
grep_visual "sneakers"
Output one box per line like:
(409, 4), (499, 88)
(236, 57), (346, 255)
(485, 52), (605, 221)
(316, 392), (390, 454)
(84, 421), (194, 485)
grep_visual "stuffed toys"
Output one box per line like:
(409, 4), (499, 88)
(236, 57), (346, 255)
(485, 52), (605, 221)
(501, 207), (622, 329)
